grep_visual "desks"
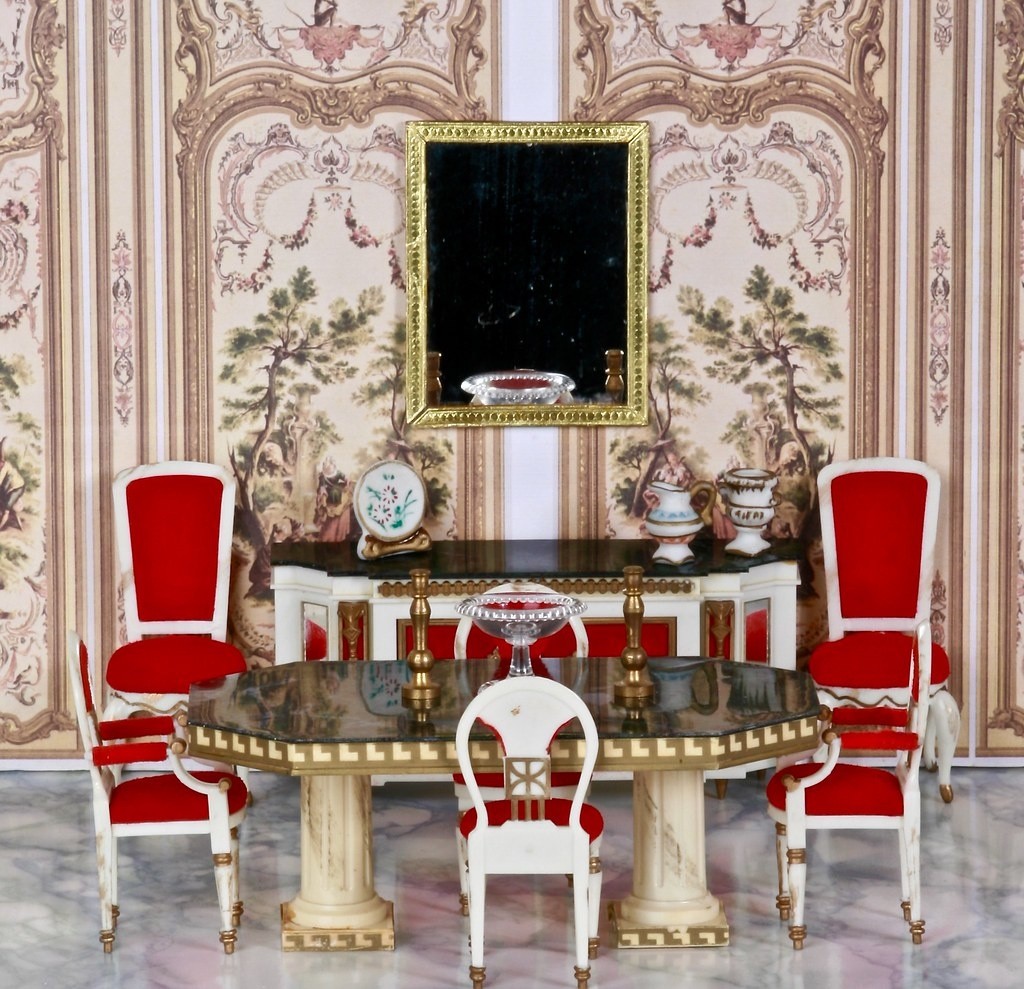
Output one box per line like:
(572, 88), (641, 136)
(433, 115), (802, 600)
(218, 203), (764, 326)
(185, 659), (821, 952)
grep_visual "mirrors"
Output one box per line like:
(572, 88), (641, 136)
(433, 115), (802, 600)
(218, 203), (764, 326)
(406, 120), (651, 429)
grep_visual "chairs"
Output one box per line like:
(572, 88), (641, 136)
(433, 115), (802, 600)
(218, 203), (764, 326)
(457, 676), (604, 989)
(767, 617), (932, 949)
(810, 456), (961, 804)
(453, 581), (589, 659)
(97, 462), (247, 774)
(65, 630), (250, 956)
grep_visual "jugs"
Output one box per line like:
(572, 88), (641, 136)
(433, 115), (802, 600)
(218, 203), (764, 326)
(642, 480), (717, 569)
(716, 468), (779, 559)
